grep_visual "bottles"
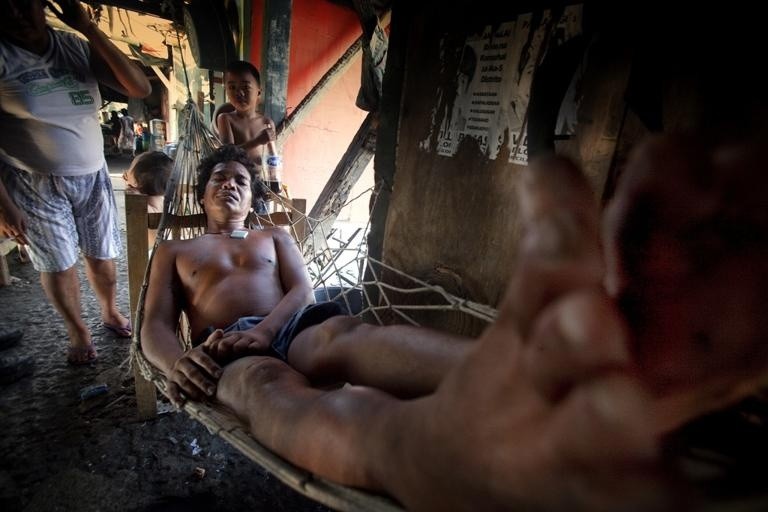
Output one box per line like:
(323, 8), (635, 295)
(260, 123), (284, 196)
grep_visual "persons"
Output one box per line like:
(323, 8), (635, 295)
(0, 1), (153, 365)
(117, 108), (136, 157)
(137, 123), (145, 136)
(217, 58), (277, 229)
(139, 144), (768, 510)
(104, 110), (120, 145)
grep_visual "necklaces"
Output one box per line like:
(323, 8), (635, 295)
(204, 229), (251, 239)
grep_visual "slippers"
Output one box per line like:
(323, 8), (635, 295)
(71, 340), (96, 364)
(104, 320), (132, 336)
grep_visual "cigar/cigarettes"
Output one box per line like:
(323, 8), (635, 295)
(3, 237), (17, 242)
